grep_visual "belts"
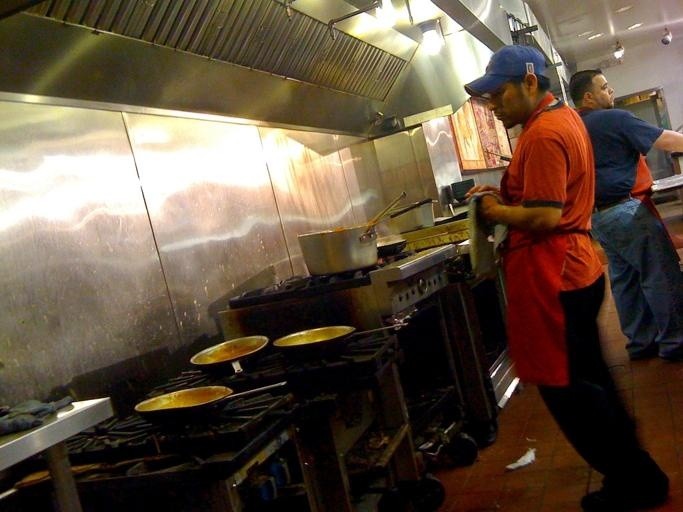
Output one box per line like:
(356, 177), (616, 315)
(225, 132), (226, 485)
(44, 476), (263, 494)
(592, 197), (629, 214)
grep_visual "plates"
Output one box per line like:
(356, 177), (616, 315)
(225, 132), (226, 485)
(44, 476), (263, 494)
(651, 173), (683, 191)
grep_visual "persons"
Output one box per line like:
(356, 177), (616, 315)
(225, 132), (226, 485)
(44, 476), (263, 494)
(463, 44), (669, 512)
(568, 68), (683, 362)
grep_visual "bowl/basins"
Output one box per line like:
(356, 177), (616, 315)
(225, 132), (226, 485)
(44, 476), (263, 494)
(451, 178), (476, 205)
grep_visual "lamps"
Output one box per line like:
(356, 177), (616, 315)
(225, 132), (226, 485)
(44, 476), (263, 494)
(612, 40), (625, 64)
(661, 27), (673, 45)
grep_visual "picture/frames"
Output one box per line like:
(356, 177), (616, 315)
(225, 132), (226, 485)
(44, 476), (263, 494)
(449, 95), (514, 172)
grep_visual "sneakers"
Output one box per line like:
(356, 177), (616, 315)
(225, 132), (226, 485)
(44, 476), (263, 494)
(580, 473), (670, 510)
(630, 340), (682, 361)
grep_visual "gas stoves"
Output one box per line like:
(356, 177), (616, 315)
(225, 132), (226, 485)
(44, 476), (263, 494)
(43, 337), (391, 487)
(218, 243), (456, 343)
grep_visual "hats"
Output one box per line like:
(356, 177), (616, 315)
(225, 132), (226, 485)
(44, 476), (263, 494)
(464, 44), (550, 97)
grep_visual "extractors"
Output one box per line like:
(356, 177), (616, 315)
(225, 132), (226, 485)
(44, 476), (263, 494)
(0, 0), (425, 137)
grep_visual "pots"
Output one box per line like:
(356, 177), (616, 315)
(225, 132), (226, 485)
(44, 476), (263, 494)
(133, 324), (410, 417)
(390, 198), (439, 234)
(377, 239), (407, 256)
(297, 191), (407, 276)
